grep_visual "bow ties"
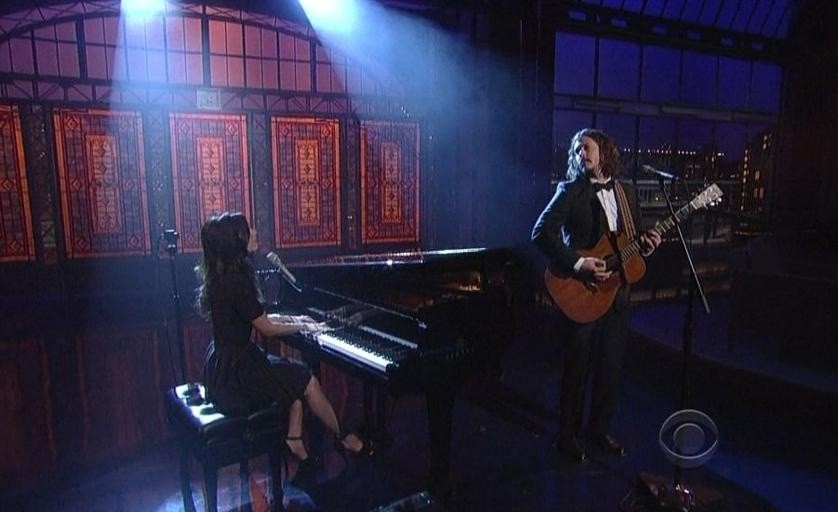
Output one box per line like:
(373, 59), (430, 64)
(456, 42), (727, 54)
(592, 180), (615, 193)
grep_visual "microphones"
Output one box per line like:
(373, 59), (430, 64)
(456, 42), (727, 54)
(643, 165), (675, 180)
(266, 251), (296, 283)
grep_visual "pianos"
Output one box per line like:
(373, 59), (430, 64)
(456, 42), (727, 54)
(265, 247), (519, 508)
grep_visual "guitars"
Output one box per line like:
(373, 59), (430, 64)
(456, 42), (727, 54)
(542, 183), (724, 327)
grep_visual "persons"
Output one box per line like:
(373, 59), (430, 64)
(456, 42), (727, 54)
(528, 126), (664, 455)
(188, 210), (381, 477)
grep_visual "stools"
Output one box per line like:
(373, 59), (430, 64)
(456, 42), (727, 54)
(162, 381), (290, 512)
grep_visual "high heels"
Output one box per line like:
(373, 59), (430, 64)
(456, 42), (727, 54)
(283, 434), (324, 471)
(332, 429), (374, 463)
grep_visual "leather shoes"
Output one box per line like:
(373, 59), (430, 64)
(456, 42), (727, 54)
(586, 427), (628, 457)
(556, 428), (591, 464)
